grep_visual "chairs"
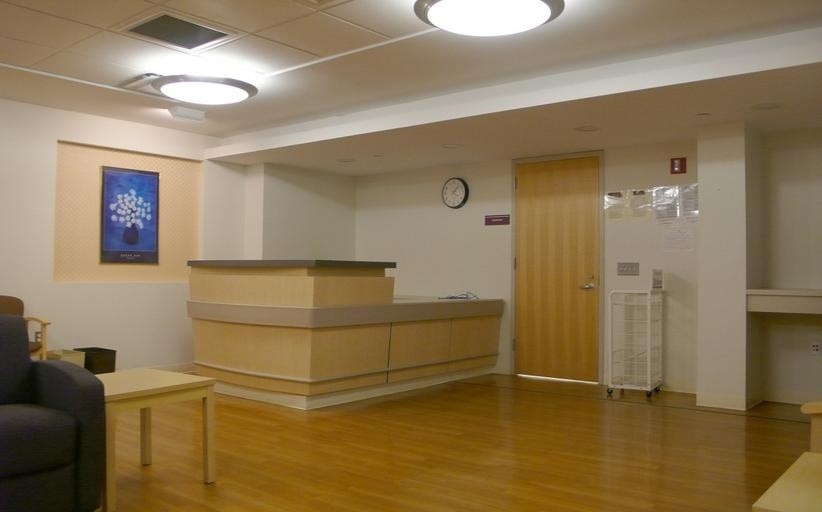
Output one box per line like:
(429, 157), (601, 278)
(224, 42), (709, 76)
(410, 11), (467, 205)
(0, 313), (106, 512)
(0, 295), (52, 360)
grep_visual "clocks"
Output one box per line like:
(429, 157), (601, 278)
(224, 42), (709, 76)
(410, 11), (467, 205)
(441, 177), (469, 209)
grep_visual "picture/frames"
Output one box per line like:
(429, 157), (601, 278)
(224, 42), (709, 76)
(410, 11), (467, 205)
(101, 166), (160, 264)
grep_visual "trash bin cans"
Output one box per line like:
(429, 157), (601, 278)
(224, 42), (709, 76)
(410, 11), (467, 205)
(39, 347), (116, 374)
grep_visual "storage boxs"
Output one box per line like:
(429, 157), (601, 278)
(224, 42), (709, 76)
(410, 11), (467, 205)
(40, 348), (86, 370)
(71, 347), (116, 374)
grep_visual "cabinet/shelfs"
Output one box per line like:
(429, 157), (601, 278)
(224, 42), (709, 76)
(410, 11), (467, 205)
(606, 287), (663, 398)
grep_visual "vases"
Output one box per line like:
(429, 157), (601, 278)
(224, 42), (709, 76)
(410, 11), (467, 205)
(123, 219), (139, 243)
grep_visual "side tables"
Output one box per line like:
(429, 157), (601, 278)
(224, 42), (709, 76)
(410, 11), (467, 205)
(93, 367), (217, 512)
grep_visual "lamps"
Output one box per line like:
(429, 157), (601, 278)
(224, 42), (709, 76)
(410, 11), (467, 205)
(151, 75), (258, 107)
(413, 0), (565, 36)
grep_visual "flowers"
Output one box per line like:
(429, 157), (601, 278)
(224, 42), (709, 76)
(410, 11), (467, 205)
(109, 187), (152, 228)
